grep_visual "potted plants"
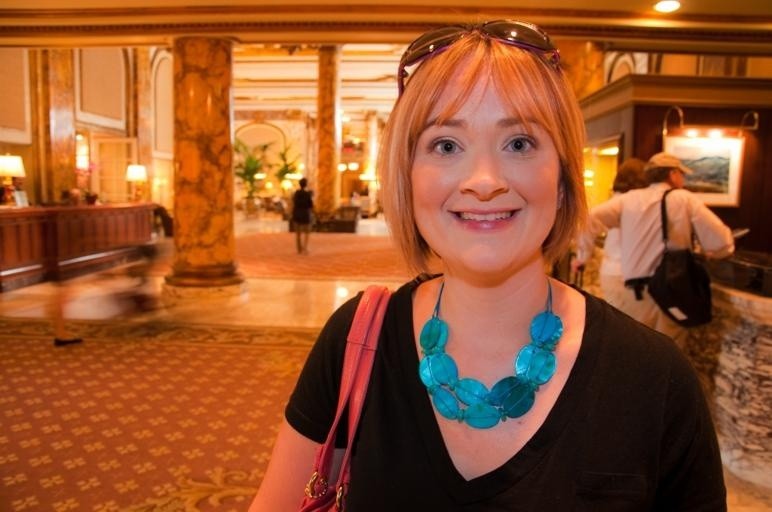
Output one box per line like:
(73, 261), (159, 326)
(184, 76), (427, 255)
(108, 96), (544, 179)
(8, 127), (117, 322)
(234, 135), (276, 221)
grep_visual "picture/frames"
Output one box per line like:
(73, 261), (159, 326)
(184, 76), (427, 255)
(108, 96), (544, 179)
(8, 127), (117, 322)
(661, 136), (746, 209)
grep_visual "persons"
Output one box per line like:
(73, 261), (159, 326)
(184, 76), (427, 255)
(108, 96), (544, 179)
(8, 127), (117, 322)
(292, 177), (313, 254)
(152, 207), (173, 238)
(67, 188), (82, 206)
(2, 177), (24, 205)
(244, 20), (728, 512)
(83, 191), (101, 205)
(569, 152), (734, 350)
(599, 158), (650, 313)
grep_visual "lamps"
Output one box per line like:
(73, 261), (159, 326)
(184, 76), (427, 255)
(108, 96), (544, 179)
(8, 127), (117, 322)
(0, 153), (27, 204)
(124, 162), (151, 205)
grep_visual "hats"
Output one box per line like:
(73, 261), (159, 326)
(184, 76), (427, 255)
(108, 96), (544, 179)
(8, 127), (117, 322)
(644, 152), (692, 177)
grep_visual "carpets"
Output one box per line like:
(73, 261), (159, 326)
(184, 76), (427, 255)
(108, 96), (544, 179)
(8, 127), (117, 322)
(101, 232), (414, 283)
(0, 319), (325, 511)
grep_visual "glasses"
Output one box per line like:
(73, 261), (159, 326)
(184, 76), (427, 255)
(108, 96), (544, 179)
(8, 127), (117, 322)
(398, 19), (559, 95)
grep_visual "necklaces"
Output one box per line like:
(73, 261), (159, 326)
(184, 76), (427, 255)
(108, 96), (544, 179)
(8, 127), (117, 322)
(418, 277), (563, 429)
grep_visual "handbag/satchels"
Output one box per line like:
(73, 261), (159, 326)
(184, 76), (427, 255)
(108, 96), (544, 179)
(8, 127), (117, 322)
(647, 249), (712, 326)
